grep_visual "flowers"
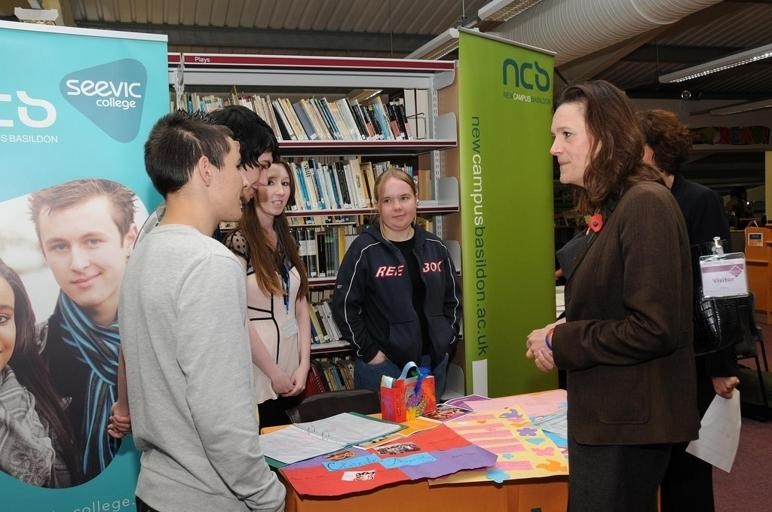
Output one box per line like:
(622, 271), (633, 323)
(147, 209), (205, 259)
(582, 207), (605, 235)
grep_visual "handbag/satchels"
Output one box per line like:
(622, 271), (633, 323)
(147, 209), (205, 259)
(695, 298), (721, 353)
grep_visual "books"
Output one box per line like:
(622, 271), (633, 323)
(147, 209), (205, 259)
(276, 154), (413, 211)
(170, 93), (413, 142)
(298, 289), (355, 400)
(420, 220), (433, 233)
(287, 216), (368, 279)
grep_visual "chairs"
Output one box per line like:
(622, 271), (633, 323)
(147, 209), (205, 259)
(733, 292), (770, 407)
(298, 387), (377, 419)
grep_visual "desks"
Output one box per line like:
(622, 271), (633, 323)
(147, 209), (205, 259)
(258, 388), (571, 511)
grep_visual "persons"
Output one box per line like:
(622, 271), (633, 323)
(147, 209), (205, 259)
(523, 78), (702, 511)
(332, 167), (461, 405)
(105, 105), (279, 439)
(27, 180), (137, 485)
(220, 157), (312, 427)
(1, 257), (82, 488)
(117, 106), (297, 512)
(633, 108), (741, 511)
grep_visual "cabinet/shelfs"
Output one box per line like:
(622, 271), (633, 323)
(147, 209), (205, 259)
(166, 51), (464, 422)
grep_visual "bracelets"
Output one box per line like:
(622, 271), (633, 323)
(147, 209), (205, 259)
(544, 326), (554, 350)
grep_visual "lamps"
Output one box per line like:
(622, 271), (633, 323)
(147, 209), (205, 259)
(478, 0), (544, 23)
(657, 42), (772, 85)
(404, 1), (482, 61)
